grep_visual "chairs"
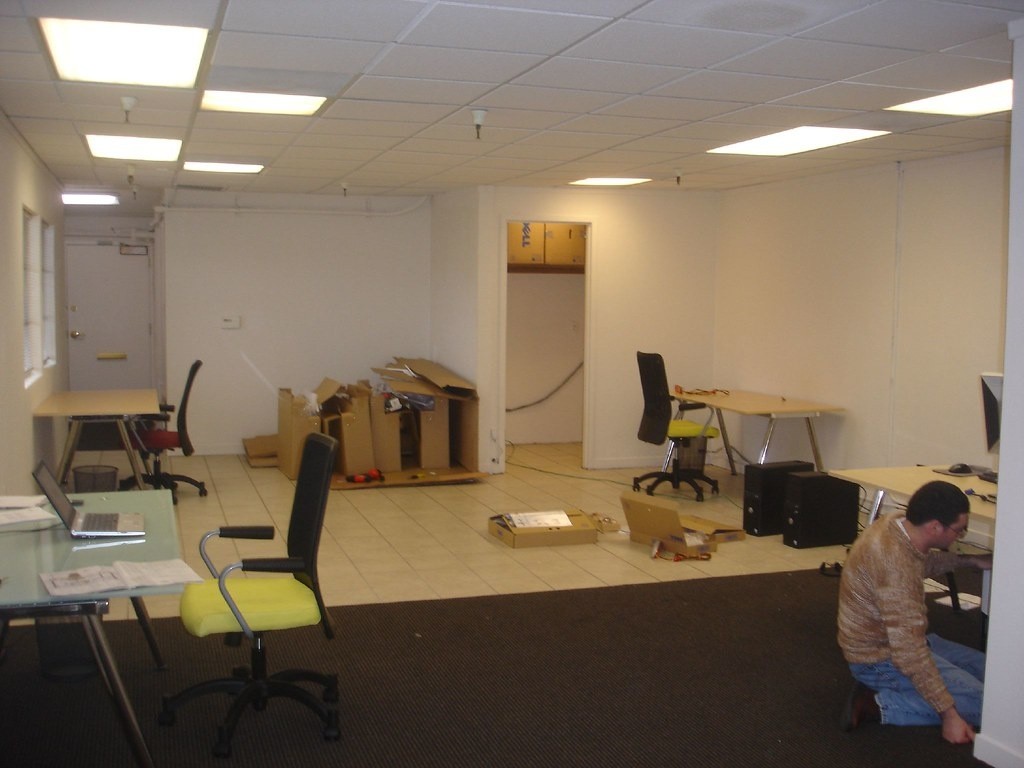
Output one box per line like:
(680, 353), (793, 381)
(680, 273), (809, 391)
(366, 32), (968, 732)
(922, 537), (990, 610)
(121, 359), (208, 504)
(158, 429), (348, 757)
(632, 350), (721, 499)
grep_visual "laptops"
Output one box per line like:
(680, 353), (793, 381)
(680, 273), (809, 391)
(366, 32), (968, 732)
(31, 459), (146, 537)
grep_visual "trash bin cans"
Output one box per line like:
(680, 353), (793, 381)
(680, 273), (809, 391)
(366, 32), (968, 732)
(74, 465), (120, 493)
(676, 438), (706, 473)
(37, 615), (102, 680)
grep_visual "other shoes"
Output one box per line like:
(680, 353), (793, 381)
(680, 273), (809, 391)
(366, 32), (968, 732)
(841, 680), (870, 733)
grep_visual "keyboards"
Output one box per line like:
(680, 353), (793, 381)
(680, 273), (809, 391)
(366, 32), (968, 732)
(978, 471), (999, 484)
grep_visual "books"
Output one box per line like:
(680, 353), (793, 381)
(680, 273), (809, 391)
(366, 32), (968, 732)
(40, 559), (204, 597)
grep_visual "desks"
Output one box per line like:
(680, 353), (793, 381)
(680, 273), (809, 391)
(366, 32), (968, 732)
(663, 388), (845, 487)
(0, 485), (188, 767)
(33, 387), (161, 491)
(828, 460), (996, 530)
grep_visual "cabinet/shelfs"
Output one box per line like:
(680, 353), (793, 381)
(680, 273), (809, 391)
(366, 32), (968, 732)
(505, 223), (586, 273)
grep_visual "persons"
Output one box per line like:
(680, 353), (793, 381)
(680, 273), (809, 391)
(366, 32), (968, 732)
(837, 481), (986, 743)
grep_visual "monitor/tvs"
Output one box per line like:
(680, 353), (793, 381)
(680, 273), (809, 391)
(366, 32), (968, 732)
(981, 373), (1004, 452)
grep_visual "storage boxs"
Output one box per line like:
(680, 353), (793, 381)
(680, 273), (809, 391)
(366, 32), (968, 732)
(489, 491), (746, 559)
(242, 356), (471, 483)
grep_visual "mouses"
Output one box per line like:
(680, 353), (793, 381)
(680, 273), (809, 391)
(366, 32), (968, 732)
(949, 463), (972, 474)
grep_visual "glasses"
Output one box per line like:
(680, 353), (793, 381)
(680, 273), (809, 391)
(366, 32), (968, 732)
(936, 520), (966, 539)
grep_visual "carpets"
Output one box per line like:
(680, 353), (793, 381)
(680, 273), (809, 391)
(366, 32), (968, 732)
(1, 561), (986, 767)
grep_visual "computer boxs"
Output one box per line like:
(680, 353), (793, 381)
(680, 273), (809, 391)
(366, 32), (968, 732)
(742, 460), (860, 550)
